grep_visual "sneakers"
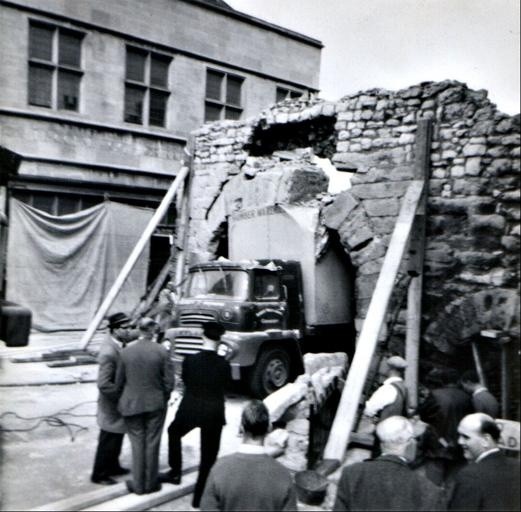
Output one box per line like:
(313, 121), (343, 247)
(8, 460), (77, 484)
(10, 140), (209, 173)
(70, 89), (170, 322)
(202, 320), (226, 341)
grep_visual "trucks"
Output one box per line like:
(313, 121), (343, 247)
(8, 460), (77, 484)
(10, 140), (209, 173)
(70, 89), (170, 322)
(161, 207), (351, 400)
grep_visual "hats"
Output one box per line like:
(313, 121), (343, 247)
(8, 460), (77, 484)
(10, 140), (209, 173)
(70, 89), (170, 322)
(387, 356), (407, 369)
(106, 312), (132, 329)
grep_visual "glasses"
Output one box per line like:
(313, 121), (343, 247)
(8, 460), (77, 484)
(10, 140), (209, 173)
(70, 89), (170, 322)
(119, 324), (135, 330)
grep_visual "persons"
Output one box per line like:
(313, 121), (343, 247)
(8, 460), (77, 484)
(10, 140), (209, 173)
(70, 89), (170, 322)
(364, 356), (408, 459)
(442, 413), (521, 511)
(200, 399), (298, 512)
(159, 322), (232, 508)
(409, 368), (471, 486)
(333, 416), (447, 512)
(92, 312), (131, 485)
(461, 369), (498, 419)
(115, 318), (175, 495)
